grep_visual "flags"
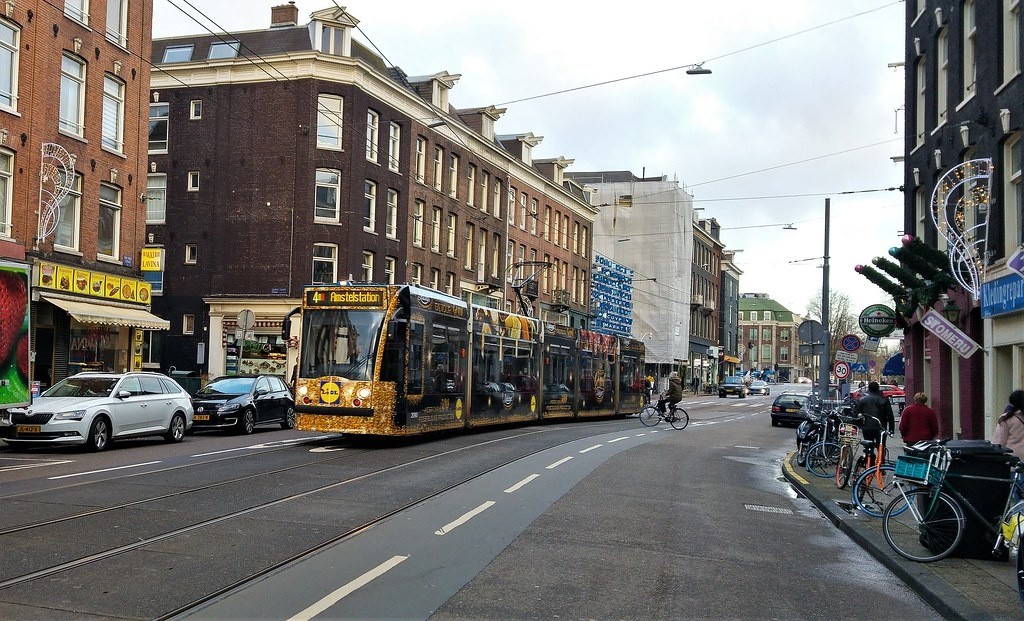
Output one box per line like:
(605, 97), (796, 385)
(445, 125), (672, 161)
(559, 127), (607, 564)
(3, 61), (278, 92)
(719, 358), (730, 366)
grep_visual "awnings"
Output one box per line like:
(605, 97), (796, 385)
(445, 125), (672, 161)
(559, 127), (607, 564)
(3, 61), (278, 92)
(883, 352), (905, 376)
(40, 293), (171, 331)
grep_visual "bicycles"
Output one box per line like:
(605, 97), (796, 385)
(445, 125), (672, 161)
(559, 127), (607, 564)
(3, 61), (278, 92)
(808, 410), (1024, 612)
(640, 390), (689, 431)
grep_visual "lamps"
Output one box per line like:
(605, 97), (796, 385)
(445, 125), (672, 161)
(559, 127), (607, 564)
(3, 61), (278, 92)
(940, 299), (964, 332)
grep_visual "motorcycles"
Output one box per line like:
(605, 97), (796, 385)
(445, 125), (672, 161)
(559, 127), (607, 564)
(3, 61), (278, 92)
(791, 399), (830, 466)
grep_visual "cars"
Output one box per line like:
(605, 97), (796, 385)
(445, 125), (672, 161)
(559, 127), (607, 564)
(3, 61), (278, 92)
(851, 384), (904, 404)
(718, 376), (770, 398)
(772, 390), (811, 427)
(192, 375), (294, 434)
(0, 372), (194, 452)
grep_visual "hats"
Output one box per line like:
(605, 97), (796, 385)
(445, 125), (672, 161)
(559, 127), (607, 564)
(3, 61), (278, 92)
(669, 371), (679, 378)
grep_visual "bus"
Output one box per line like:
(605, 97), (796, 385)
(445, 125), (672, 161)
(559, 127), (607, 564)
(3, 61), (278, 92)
(280, 281), (646, 439)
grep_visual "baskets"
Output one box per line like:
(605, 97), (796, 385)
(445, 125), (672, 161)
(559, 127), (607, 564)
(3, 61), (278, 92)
(894, 455), (945, 487)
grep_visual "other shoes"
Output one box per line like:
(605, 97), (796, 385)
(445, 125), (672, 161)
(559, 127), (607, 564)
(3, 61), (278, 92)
(656, 414), (667, 418)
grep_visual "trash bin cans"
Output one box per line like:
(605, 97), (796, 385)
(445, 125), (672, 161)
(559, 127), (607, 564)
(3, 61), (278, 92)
(902, 438), (1021, 562)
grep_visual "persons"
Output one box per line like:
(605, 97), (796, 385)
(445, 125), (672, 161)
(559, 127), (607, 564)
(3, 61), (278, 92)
(654, 373), (658, 394)
(647, 375), (654, 396)
(658, 372), (682, 417)
(853, 382), (894, 475)
(858, 380), (898, 388)
(290, 357), (298, 388)
(645, 377), (651, 404)
(693, 376), (699, 394)
(991, 390), (1024, 504)
(899, 392), (939, 448)
(349, 344), (366, 365)
